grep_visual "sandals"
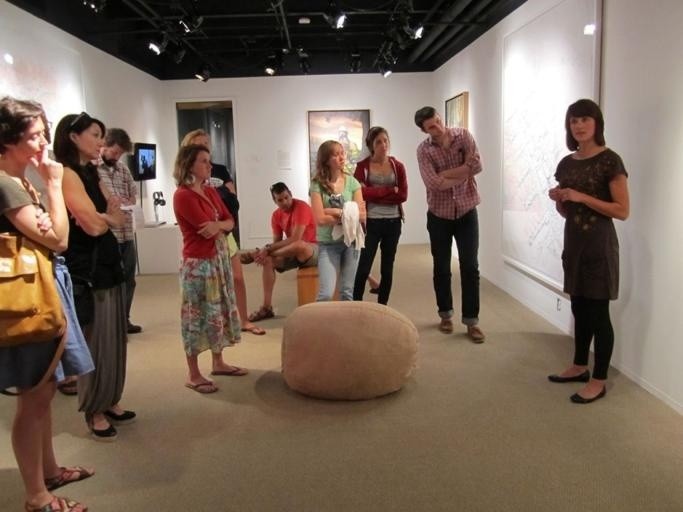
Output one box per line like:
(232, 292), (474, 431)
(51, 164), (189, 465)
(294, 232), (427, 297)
(240, 248), (259, 264)
(44, 465), (95, 490)
(26, 495), (88, 512)
(248, 305), (272, 322)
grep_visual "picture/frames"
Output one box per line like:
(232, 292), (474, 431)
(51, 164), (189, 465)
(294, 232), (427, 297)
(308, 109), (370, 180)
(445, 92), (468, 130)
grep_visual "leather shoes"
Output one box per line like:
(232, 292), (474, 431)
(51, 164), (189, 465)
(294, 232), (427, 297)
(127, 320), (140, 333)
(548, 370), (589, 382)
(468, 325), (485, 343)
(104, 409), (136, 425)
(439, 317), (453, 334)
(86, 411), (120, 442)
(570, 384), (605, 403)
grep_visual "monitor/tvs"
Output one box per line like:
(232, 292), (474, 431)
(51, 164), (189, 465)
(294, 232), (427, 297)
(132, 142), (159, 182)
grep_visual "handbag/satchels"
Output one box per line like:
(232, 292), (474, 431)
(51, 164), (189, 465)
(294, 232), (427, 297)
(0, 231), (67, 348)
(329, 193), (344, 225)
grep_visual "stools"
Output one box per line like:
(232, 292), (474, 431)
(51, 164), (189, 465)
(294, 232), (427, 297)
(281, 300), (419, 400)
(295, 267), (339, 306)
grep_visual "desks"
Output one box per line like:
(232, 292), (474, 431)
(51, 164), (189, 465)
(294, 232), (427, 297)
(135, 222), (183, 275)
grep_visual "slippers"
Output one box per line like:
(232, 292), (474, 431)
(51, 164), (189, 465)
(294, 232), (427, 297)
(370, 288), (379, 293)
(57, 381), (77, 395)
(211, 366), (248, 376)
(241, 324), (265, 334)
(184, 380), (218, 393)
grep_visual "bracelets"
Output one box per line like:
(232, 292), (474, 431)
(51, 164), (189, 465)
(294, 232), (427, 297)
(265, 243), (273, 253)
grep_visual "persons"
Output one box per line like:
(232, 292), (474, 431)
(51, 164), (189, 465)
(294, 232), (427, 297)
(53, 113), (137, 441)
(180, 129), (267, 335)
(307, 141), (366, 300)
(239, 183), (317, 321)
(365, 273), (382, 294)
(20, 100), (78, 396)
(547, 99), (629, 404)
(415, 105), (486, 344)
(92, 129), (142, 334)
(1, 94), (95, 512)
(353, 126), (408, 306)
(173, 144), (248, 394)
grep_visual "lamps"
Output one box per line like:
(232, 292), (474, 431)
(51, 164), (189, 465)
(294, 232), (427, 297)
(380, 58), (392, 78)
(321, 6), (346, 30)
(402, 15), (423, 39)
(180, 12), (203, 32)
(148, 29), (167, 54)
(85, 0), (114, 13)
(196, 56), (217, 82)
(264, 53), (282, 77)
(152, 191), (166, 223)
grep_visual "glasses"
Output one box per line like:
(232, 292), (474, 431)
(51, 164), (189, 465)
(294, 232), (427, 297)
(70, 112), (88, 128)
